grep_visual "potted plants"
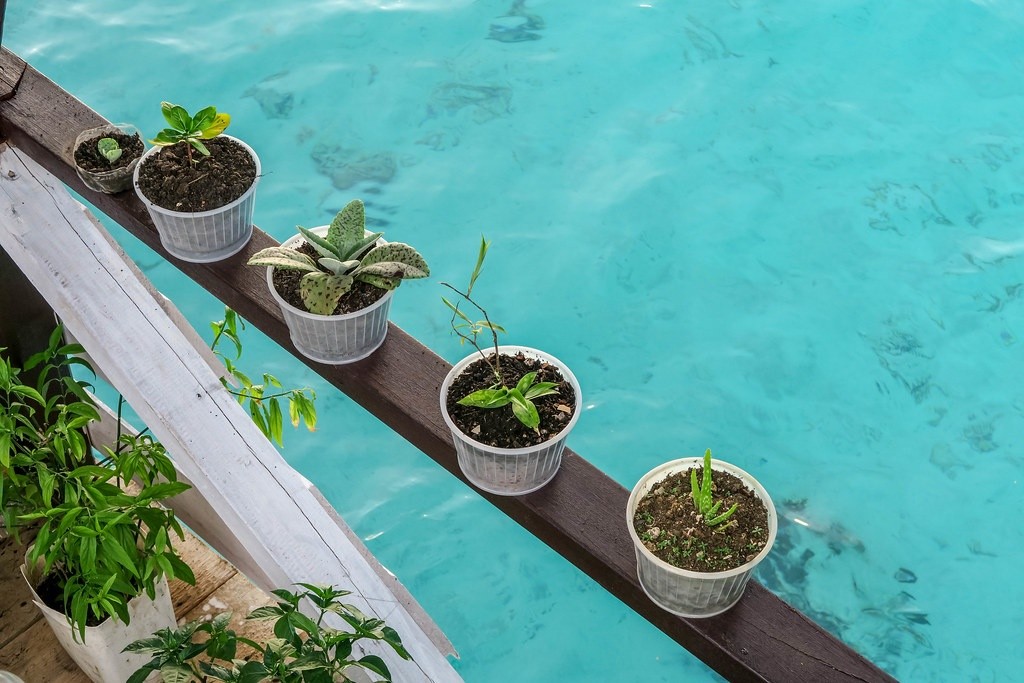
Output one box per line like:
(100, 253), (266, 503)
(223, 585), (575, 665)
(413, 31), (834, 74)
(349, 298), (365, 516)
(132, 100), (261, 264)
(626, 447), (778, 617)
(438, 233), (583, 496)
(247, 199), (430, 365)
(0, 305), (317, 683)
(72, 121), (146, 194)
